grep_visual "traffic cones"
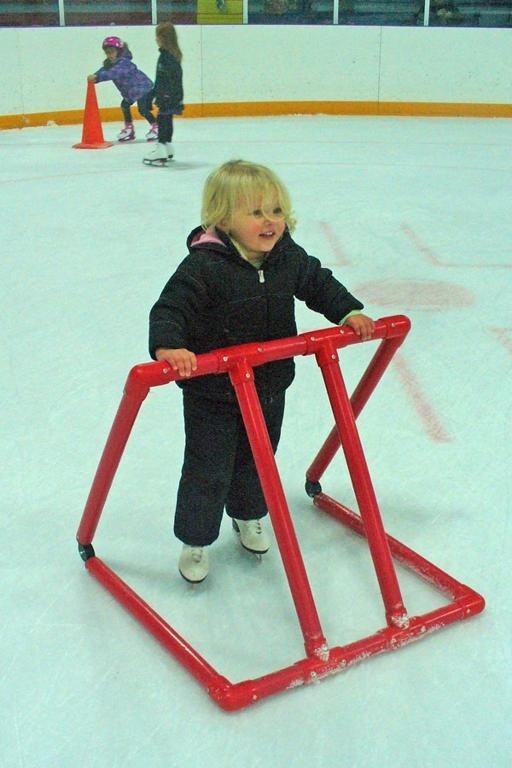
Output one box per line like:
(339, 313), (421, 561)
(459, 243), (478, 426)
(71, 79), (114, 150)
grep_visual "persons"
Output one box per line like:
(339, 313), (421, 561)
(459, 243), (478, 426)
(147, 158), (377, 585)
(415, 1), (463, 27)
(87, 36), (159, 141)
(143, 22), (185, 161)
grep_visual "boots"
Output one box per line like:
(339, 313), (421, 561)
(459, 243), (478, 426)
(118, 122), (134, 138)
(143, 142), (173, 162)
(232, 517), (272, 553)
(179, 543), (210, 584)
(145, 122), (159, 139)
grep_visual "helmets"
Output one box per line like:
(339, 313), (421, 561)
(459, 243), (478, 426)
(103, 36), (124, 51)
